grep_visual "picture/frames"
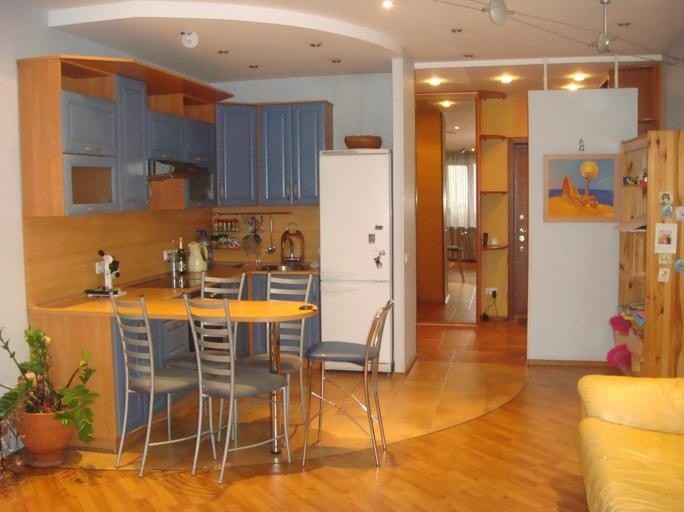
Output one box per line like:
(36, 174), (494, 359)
(543, 153), (617, 225)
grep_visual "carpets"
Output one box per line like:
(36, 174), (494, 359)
(60, 360), (528, 472)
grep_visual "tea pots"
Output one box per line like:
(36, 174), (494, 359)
(186, 241), (209, 272)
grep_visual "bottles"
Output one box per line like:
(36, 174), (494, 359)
(177, 236), (186, 275)
(213, 218), (240, 231)
(196, 229), (214, 269)
(168, 239), (180, 280)
(219, 234), (228, 245)
(483, 231), (489, 246)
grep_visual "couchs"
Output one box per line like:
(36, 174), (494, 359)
(576, 374), (684, 512)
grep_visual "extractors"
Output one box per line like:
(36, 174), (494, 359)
(148, 159), (214, 198)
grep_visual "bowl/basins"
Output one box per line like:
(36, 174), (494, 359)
(344, 136), (382, 149)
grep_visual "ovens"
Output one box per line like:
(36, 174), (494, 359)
(189, 286), (224, 352)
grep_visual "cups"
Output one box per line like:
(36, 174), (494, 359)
(492, 237), (498, 246)
(255, 256), (264, 266)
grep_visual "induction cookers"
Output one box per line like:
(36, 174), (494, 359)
(128, 276), (208, 289)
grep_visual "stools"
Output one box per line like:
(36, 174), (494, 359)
(447, 245), (465, 283)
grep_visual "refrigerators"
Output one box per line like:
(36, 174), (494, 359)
(319, 148), (417, 374)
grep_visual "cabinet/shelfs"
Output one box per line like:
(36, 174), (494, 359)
(257, 100), (333, 206)
(618, 127), (683, 377)
(476, 92), (512, 323)
(16, 54), (234, 218)
(216, 102), (259, 207)
(28, 314), (190, 453)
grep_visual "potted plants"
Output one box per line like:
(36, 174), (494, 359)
(0, 326), (99, 469)
